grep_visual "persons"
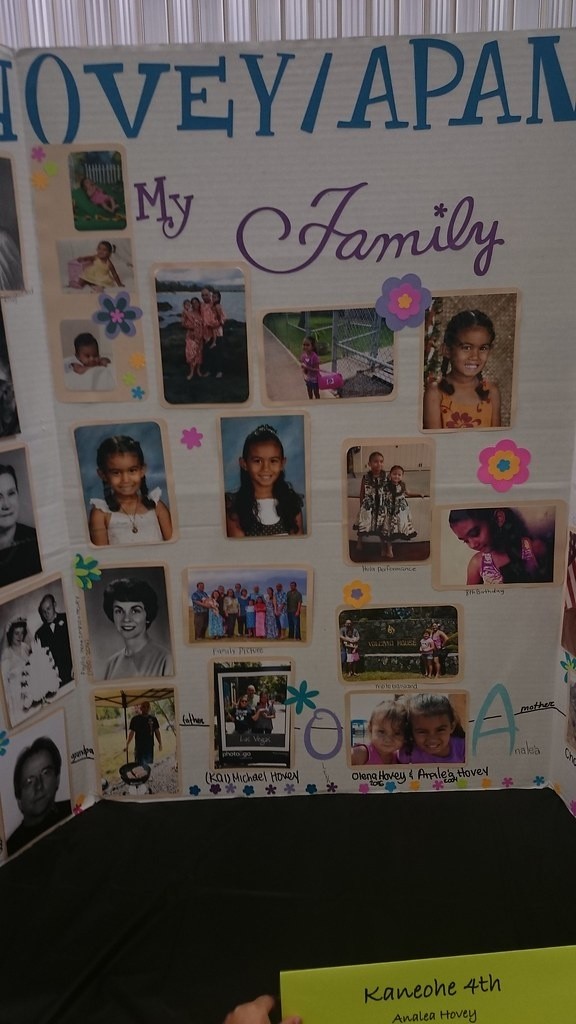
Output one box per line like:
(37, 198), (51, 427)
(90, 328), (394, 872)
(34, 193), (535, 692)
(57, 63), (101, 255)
(224, 424), (304, 538)
(223, 996), (304, 1024)
(0, 464), (43, 588)
(227, 697), (267, 733)
(62, 331), (111, 375)
(33, 592), (75, 699)
(352, 451), (388, 558)
(449, 507), (553, 586)
(0, 229), (24, 291)
(67, 240), (126, 290)
(209, 289), (223, 348)
(80, 177), (121, 213)
(121, 702), (163, 765)
(237, 685), (259, 709)
(102, 577), (173, 680)
(298, 336), (323, 399)
(191, 581), (302, 641)
(351, 694), (412, 765)
(181, 299), (200, 336)
(423, 309), (502, 429)
(251, 688), (276, 734)
(185, 296), (205, 381)
(200, 287), (226, 376)
(375, 464), (430, 558)
(396, 694), (465, 763)
(338, 618), (449, 680)
(5, 736), (73, 859)
(88, 434), (172, 547)
(0, 616), (46, 727)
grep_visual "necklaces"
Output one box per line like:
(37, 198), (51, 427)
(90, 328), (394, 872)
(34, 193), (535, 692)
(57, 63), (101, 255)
(115, 495), (140, 534)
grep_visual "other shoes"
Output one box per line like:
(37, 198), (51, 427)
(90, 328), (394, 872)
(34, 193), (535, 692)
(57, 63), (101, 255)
(186, 374), (193, 379)
(348, 671), (357, 676)
(421, 674), (444, 678)
(205, 337), (211, 342)
(204, 372), (211, 376)
(195, 634), (301, 641)
(198, 373), (202, 376)
(216, 372), (224, 378)
(209, 344), (217, 348)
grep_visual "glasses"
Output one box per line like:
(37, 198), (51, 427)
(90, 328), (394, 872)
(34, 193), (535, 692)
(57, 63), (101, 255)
(241, 701), (248, 702)
(22, 766), (55, 788)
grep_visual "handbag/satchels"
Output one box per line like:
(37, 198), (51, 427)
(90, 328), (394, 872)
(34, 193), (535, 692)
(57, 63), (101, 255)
(318, 370), (344, 390)
